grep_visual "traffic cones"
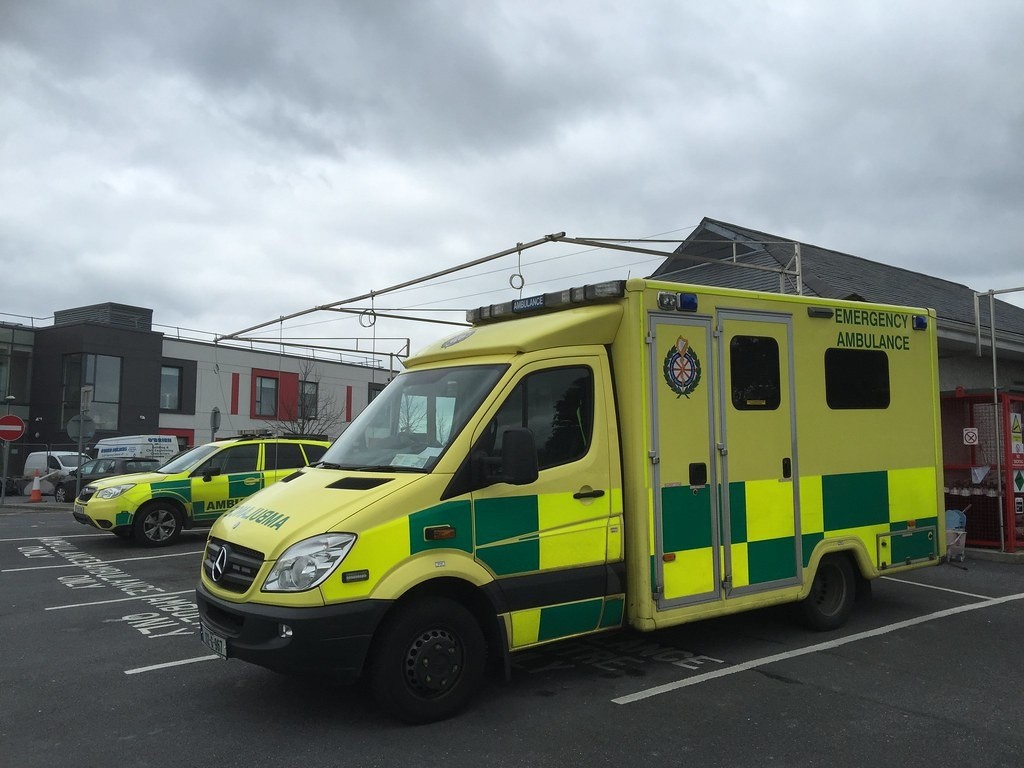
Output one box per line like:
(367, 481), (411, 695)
(23, 469), (51, 504)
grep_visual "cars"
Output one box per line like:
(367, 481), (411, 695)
(53, 457), (161, 504)
(73, 433), (336, 548)
(23, 451), (104, 483)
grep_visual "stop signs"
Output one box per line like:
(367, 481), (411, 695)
(0, 415), (25, 443)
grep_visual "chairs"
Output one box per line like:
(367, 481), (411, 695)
(451, 410), (498, 455)
(529, 400), (584, 467)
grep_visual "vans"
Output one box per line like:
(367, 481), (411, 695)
(89, 434), (180, 471)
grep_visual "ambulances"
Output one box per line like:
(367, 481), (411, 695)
(194, 276), (952, 724)
